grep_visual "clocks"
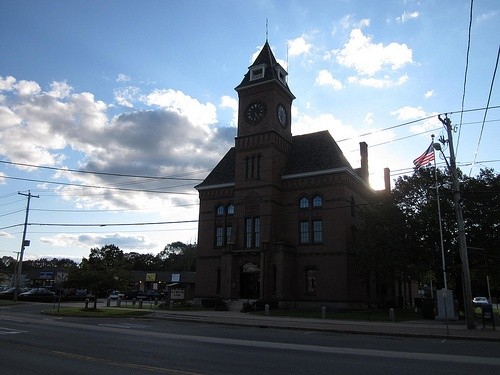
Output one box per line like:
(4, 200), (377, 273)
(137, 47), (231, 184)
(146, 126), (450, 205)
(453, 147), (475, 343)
(245, 100), (267, 126)
(277, 103), (288, 129)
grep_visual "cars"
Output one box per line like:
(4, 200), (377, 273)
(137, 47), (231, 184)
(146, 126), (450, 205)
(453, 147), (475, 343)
(472, 297), (489, 308)
(0, 284), (125, 300)
